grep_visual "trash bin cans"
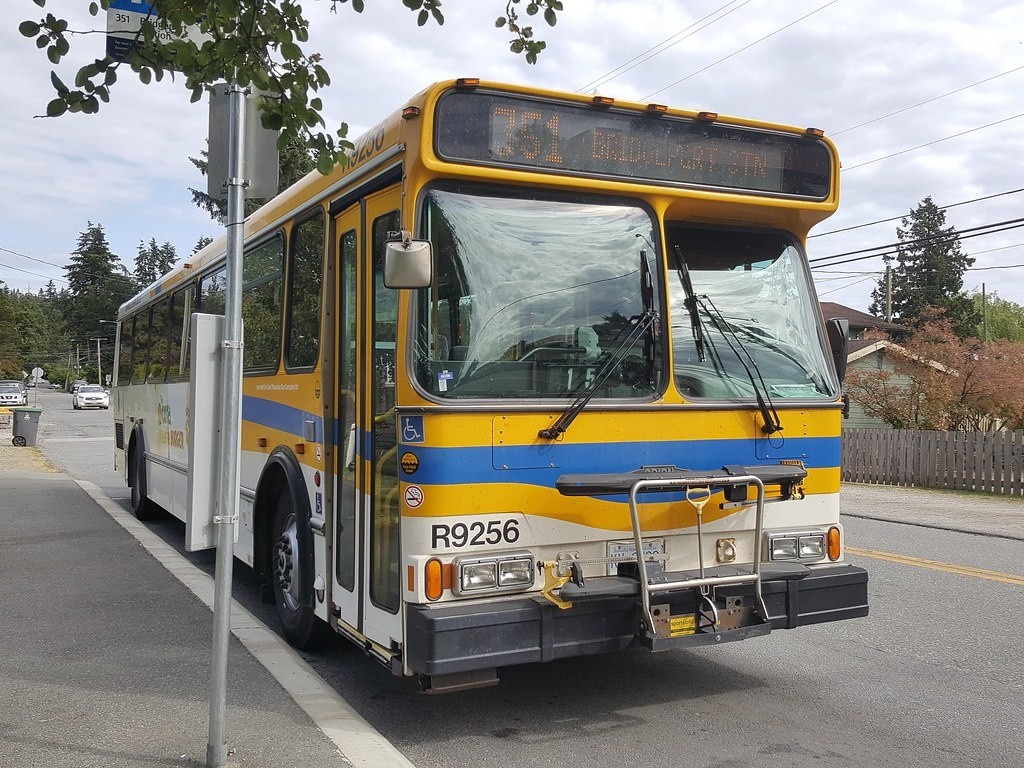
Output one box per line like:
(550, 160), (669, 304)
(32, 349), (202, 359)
(7, 407), (44, 447)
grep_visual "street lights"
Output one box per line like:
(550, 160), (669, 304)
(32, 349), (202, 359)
(99, 319), (121, 388)
(88, 338), (108, 388)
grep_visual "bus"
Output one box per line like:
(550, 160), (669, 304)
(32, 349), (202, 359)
(111, 78), (871, 693)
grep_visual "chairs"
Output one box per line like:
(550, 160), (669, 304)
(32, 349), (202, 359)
(438, 334), (449, 360)
(447, 346), (481, 363)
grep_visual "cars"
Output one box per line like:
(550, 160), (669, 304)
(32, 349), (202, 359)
(0, 379), (29, 406)
(69, 379), (111, 410)
(48, 383), (63, 389)
